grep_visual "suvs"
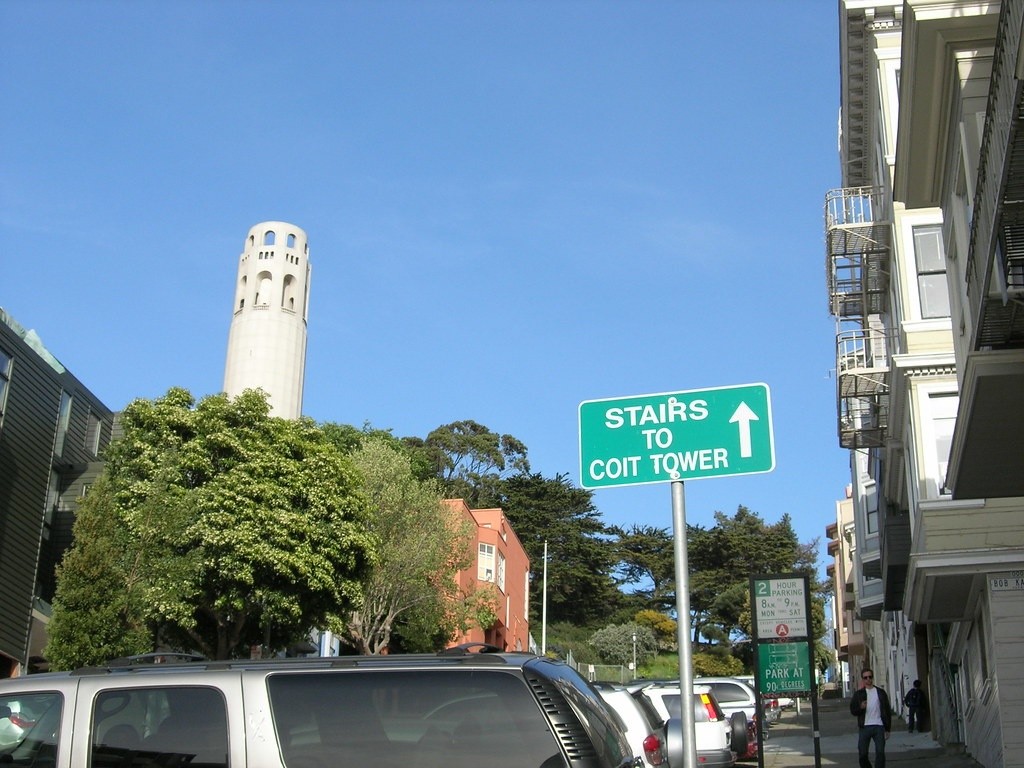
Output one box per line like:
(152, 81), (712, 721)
(623, 685), (748, 768)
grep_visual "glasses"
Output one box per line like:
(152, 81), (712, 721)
(864, 676), (873, 679)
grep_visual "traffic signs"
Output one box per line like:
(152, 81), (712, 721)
(580, 385), (775, 490)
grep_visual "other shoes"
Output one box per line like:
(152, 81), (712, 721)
(909, 730), (913, 733)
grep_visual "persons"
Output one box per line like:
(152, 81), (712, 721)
(905, 679), (927, 733)
(850, 669), (892, 768)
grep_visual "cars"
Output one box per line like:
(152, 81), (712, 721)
(425, 680), (683, 768)
(0, 700), (38, 751)
(628, 676), (765, 725)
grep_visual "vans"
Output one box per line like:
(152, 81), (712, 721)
(0, 652), (645, 768)
(733, 676), (781, 724)
